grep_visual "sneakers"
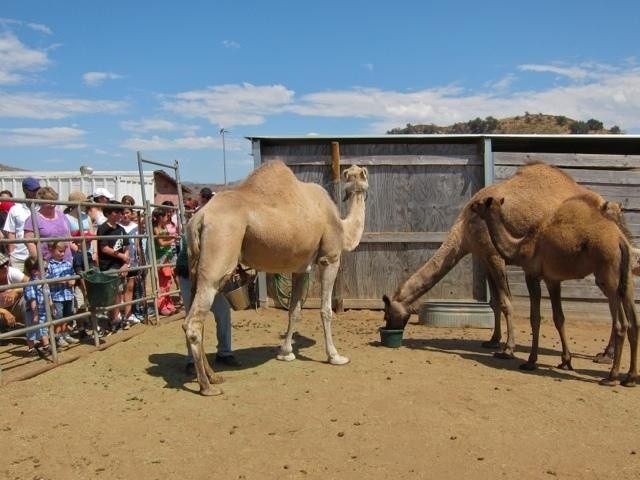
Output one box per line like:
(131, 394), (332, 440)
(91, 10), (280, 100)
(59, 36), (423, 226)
(56, 336), (68, 346)
(186, 363), (196, 375)
(63, 332), (79, 343)
(80, 311), (157, 340)
(217, 353), (241, 366)
(28, 343), (52, 359)
(159, 306), (176, 316)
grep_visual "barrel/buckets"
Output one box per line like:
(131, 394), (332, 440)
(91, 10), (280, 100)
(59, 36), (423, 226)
(377, 326), (405, 349)
(80, 268), (119, 307)
(226, 275), (253, 311)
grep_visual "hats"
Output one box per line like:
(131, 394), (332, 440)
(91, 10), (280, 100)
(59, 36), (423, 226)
(93, 188), (114, 199)
(201, 188), (211, 196)
(71, 230), (94, 242)
(0, 253), (8, 266)
(23, 177), (40, 191)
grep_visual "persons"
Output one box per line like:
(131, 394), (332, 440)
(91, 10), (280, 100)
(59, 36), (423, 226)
(0, 177), (214, 355)
